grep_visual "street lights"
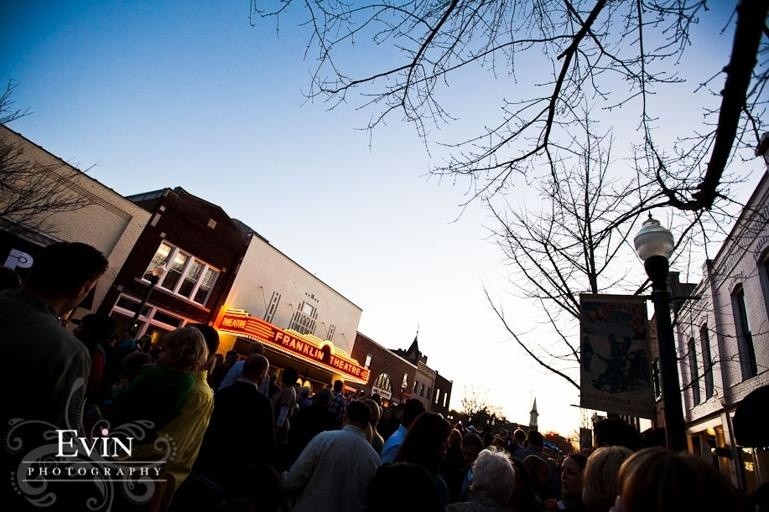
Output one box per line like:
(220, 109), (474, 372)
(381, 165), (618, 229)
(120, 258), (167, 338)
(629, 209), (692, 456)
